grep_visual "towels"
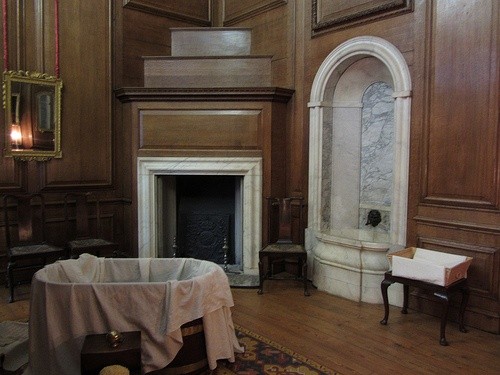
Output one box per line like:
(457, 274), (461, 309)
(56, 253), (101, 284)
(391, 248), (468, 286)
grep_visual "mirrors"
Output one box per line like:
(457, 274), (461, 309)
(2, 70), (62, 158)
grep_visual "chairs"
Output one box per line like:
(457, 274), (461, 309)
(257, 194), (310, 297)
(4, 192), (64, 303)
(63, 191), (113, 259)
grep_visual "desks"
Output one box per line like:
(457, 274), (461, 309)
(380, 270), (469, 346)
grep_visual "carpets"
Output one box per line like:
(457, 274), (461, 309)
(202, 323), (342, 375)
